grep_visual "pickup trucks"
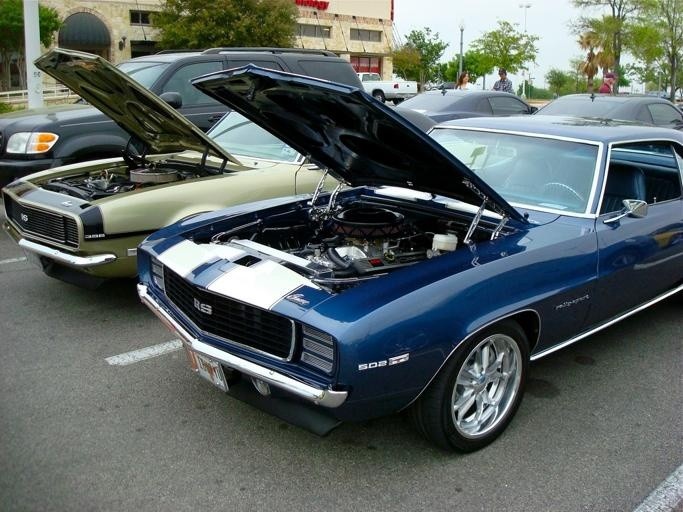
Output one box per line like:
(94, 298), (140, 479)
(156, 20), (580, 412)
(356, 72), (418, 105)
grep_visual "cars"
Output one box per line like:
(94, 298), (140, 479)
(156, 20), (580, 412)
(392, 84), (537, 135)
(134, 63), (682, 453)
(2, 48), (349, 292)
(533, 92), (682, 130)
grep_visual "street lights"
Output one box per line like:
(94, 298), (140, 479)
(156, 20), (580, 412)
(518, 1), (532, 99)
(454, 18), (467, 90)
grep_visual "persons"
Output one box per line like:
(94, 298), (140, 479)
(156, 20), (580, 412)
(599, 73), (617, 93)
(454, 71), (477, 91)
(490, 68), (515, 95)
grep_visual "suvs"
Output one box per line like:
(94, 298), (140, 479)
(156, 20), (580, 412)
(0, 44), (364, 193)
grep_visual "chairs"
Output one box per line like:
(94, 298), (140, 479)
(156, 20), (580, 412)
(498, 153), (681, 215)
